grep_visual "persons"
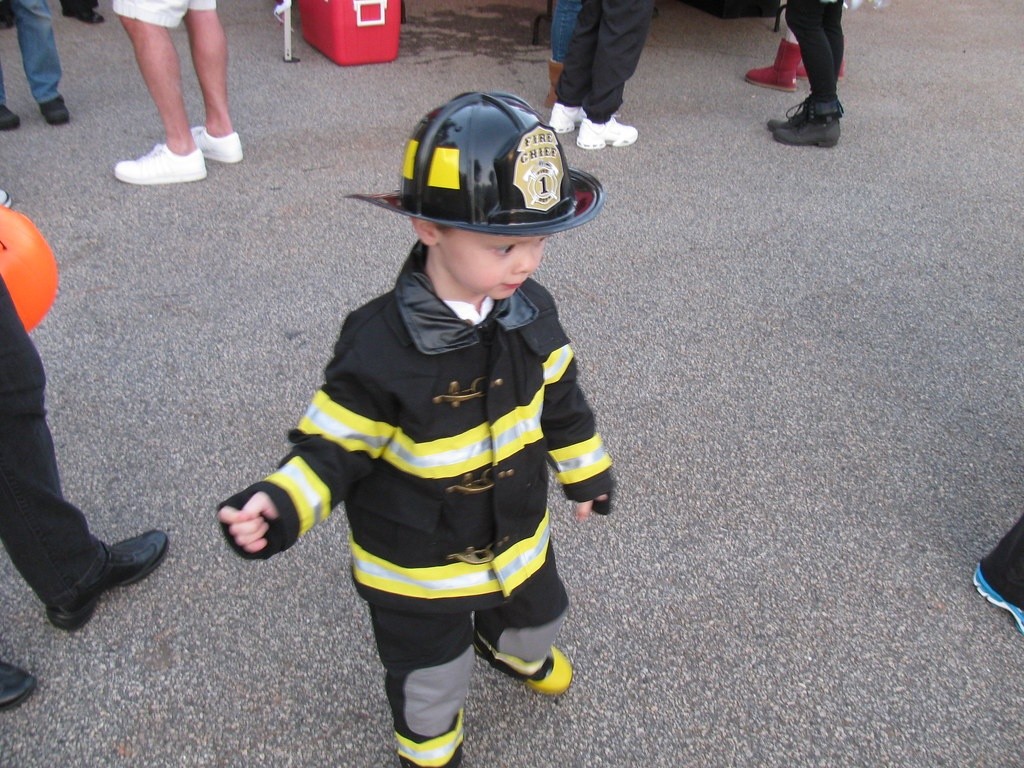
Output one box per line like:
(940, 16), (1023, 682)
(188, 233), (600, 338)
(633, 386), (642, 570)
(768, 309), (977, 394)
(973, 515), (1024, 637)
(745, 0), (846, 148)
(113, 0), (242, 184)
(547, 0), (654, 149)
(217, 90), (618, 768)
(0, 281), (169, 714)
(0, 0), (104, 131)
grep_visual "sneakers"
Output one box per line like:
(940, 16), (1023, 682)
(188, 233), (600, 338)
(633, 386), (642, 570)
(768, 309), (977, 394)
(0, 189), (11, 209)
(191, 126), (242, 163)
(973, 564), (1024, 636)
(576, 118), (638, 150)
(549, 101), (587, 134)
(114, 145), (207, 185)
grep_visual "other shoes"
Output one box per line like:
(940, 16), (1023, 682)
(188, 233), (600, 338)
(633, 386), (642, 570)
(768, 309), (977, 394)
(474, 629), (573, 695)
(395, 709), (464, 768)
(0, 104), (20, 130)
(40, 96), (69, 125)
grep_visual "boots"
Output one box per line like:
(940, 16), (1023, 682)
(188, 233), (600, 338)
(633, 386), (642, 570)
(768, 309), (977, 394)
(745, 39), (801, 92)
(768, 94), (844, 147)
(546, 58), (563, 107)
(796, 59), (846, 81)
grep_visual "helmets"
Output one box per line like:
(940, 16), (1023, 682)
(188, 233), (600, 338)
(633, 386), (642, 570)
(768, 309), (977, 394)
(344, 91), (605, 237)
(0, 206), (58, 333)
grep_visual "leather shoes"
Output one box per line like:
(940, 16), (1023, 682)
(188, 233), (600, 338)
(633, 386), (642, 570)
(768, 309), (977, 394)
(63, 9), (104, 23)
(0, 662), (37, 711)
(46, 530), (169, 629)
